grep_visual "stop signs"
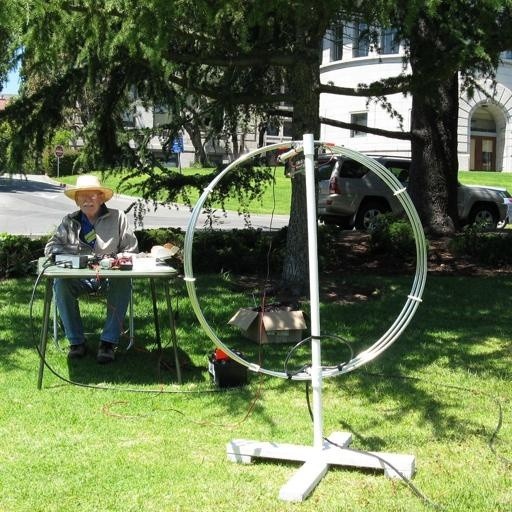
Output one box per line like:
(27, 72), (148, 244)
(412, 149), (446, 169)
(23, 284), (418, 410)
(54, 145), (65, 159)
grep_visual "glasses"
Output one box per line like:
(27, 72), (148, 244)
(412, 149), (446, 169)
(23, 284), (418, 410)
(75, 192), (101, 201)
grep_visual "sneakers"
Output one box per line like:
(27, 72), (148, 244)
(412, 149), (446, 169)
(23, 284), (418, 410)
(96, 339), (115, 364)
(68, 342), (86, 358)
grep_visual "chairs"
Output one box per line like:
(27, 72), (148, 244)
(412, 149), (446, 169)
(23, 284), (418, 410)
(52, 239), (138, 353)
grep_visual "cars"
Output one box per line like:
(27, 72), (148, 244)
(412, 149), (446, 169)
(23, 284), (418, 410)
(317, 153), (511, 233)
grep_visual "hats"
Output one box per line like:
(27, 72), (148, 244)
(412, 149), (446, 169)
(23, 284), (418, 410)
(64, 176), (113, 203)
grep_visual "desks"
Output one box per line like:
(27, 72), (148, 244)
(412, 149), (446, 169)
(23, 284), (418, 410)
(37, 253), (184, 391)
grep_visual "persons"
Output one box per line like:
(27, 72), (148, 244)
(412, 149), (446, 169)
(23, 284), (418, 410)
(44, 190), (139, 363)
